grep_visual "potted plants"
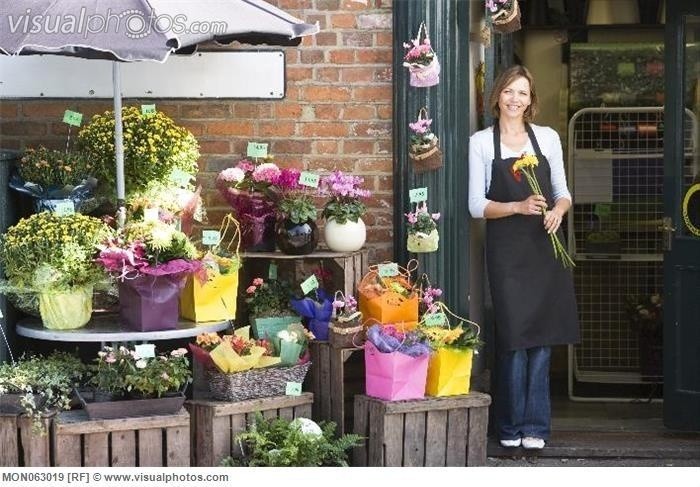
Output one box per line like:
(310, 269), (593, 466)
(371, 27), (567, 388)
(1, 347), (84, 441)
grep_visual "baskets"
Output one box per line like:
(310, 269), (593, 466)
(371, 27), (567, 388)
(200, 355), (311, 401)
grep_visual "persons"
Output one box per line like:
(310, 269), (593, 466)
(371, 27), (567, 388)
(467, 64), (573, 450)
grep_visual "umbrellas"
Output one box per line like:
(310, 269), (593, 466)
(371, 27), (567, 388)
(0, 0), (320, 231)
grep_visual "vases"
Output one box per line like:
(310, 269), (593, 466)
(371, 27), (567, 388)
(323, 212), (368, 253)
(207, 362), (313, 400)
(76, 390), (187, 420)
(33, 196), (77, 217)
(141, 206), (183, 233)
(0, 389), (53, 419)
(249, 311), (303, 342)
(240, 211), (276, 250)
(277, 213), (319, 257)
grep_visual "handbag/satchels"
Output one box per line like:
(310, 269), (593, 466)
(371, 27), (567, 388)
(351, 318), (429, 407)
(321, 289), (366, 348)
(120, 262), (188, 332)
(358, 259), (424, 331)
(419, 302), (482, 408)
(180, 212), (243, 327)
(483, 1), (524, 34)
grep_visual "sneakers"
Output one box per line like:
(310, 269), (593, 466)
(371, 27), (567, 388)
(502, 429), (546, 452)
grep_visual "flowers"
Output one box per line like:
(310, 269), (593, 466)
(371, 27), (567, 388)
(141, 170), (206, 226)
(421, 284), (443, 317)
(422, 321), (484, 351)
(218, 158), (281, 197)
(365, 322), (429, 358)
(404, 201), (442, 236)
(92, 347), (192, 401)
(243, 275), (291, 315)
(401, 37), (434, 69)
(511, 152), (577, 270)
(485, 0), (514, 21)
(339, 296), (360, 326)
(112, 219), (201, 267)
(194, 244), (242, 277)
(317, 167), (373, 224)
(271, 169), (324, 225)
(192, 324), (315, 376)
(78, 107), (201, 207)
(16, 143), (100, 191)
(0, 211), (115, 287)
(407, 118), (439, 154)
